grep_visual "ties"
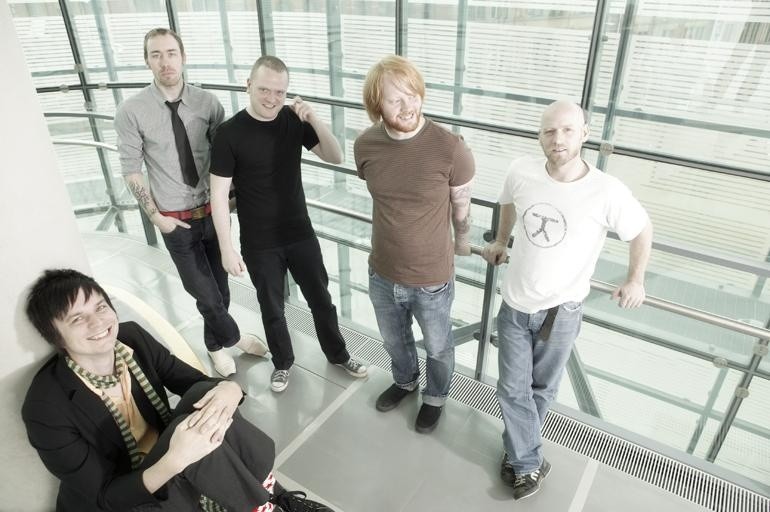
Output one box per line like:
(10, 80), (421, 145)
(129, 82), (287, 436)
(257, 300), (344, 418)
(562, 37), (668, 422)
(165, 100), (199, 188)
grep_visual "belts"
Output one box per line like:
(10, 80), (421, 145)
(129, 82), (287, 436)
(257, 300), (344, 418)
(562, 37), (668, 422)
(160, 202), (213, 222)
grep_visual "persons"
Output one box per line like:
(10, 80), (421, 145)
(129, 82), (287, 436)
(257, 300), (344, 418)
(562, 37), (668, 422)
(353, 55), (476, 433)
(22, 269), (333, 511)
(482, 100), (653, 501)
(114, 29), (269, 378)
(209, 57), (368, 392)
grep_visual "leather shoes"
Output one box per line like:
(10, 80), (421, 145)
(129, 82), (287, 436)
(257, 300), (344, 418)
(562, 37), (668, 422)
(208, 347), (236, 378)
(244, 333), (274, 361)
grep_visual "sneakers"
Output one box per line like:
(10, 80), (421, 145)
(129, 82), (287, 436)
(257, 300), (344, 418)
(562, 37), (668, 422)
(269, 480), (335, 512)
(376, 383), (419, 412)
(271, 370), (290, 392)
(415, 402), (443, 433)
(512, 459), (550, 501)
(499, 452), (515, 485)
(335, 356), (368, 377)
